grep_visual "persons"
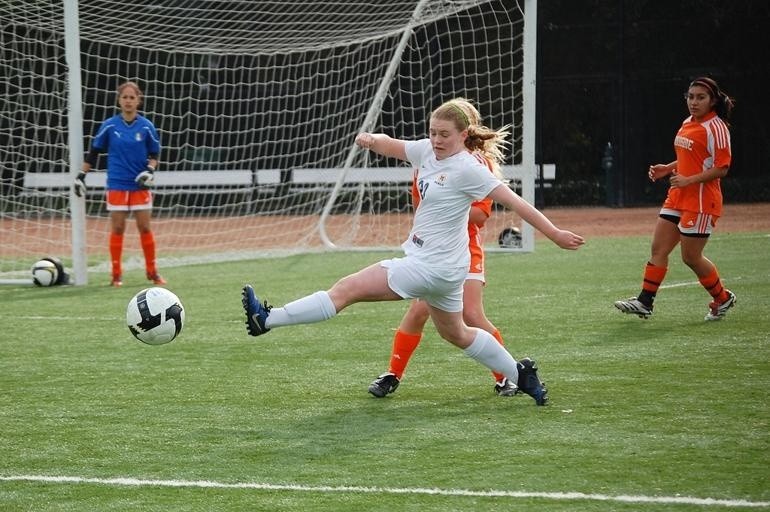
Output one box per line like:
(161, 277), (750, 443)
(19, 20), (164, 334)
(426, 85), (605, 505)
(75, 81), (166, 287)
(614, 77), (737, 319)
(369, 96), (518, 400)
(240, 101), (585, 407)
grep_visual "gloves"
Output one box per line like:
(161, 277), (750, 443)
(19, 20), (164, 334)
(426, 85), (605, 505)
(134, 164), (155, 187)
(74, 170), (87, 199)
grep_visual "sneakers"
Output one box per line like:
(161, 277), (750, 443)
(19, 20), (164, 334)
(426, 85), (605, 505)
(704, 290), (736, 321)
(368, 371), (401, 397)
(614, 297), (654, 318)
(241, 284), (273, 337)
(517, 357), (548, 406)
(111, 275), (123, 286)
(493, 376), (520, 397)
(147, 273), (167, 285)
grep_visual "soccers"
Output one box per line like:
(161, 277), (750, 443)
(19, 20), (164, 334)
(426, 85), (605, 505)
(125, 288), (186, 345)
(30, 259), (58, 286)
